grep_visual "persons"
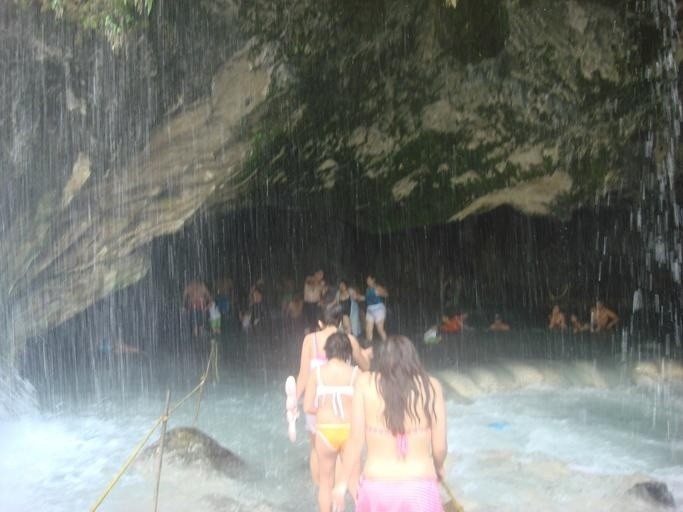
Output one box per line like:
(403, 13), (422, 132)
(302, 332), (368, 511)
(333, 336), (447, 512)
(286, 299), (373, 483)
(177, 270), (391, 362)
(421, 302), (514, 345)
(546, 299), (620, 335)
